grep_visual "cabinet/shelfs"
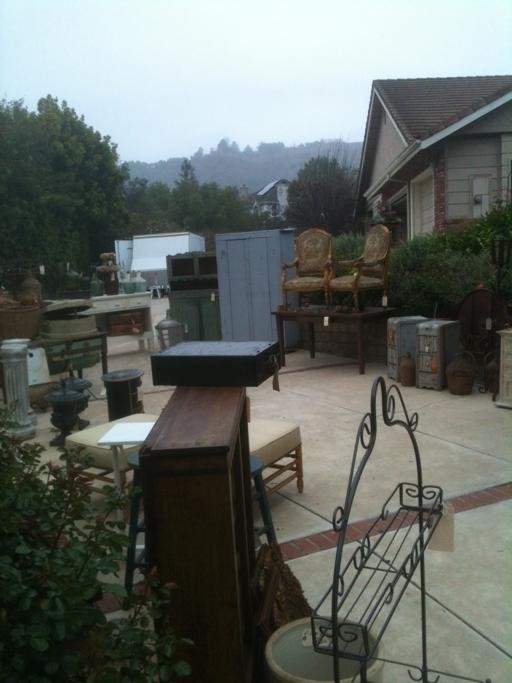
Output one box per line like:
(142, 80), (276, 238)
(166, 251), (221, 341)
(215, 228), (299, 353)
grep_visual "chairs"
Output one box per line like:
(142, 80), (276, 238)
(281, 229), (333, 311)
(327, 223), (390, 313)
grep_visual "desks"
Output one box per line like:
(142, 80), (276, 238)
(97, 423), (156, 522)
(270, 305), (398, 374)
(42, 290), (155, 354)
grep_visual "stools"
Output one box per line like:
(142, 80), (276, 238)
(66, 413), (161, 522)
(247, 417), (303, 501)
